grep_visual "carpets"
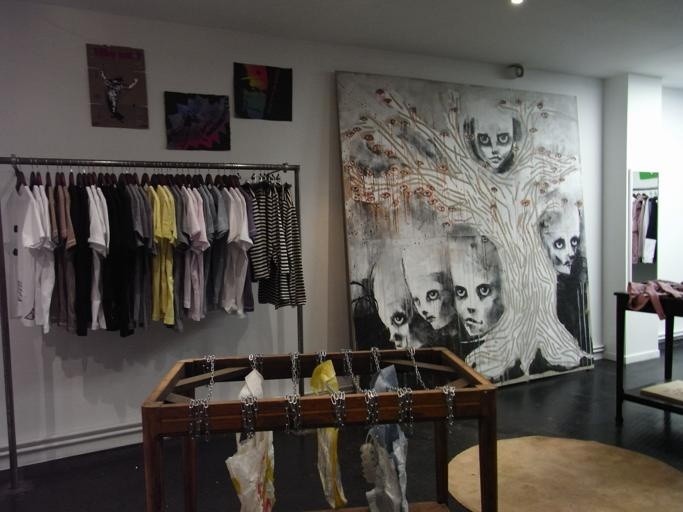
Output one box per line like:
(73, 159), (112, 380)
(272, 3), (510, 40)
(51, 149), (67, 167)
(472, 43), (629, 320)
(446, 433), (683, 512)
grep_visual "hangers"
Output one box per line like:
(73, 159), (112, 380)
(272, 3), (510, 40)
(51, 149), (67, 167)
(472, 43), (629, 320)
(10, 155), (290, 191)
(633, 184), (657, 199)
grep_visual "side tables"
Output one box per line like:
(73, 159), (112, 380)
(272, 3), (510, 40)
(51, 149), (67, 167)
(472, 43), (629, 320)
(613, 290), (683, 425)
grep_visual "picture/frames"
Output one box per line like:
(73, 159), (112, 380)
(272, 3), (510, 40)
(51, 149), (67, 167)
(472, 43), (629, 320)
(229, 60), (296, 123)
(162, 88), (231, 152)
(84, 40), (149, 132)
(334, 69), (596, 389)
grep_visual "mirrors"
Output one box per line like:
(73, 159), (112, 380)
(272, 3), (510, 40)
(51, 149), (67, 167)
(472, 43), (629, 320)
(627, 170), (659, 282)
